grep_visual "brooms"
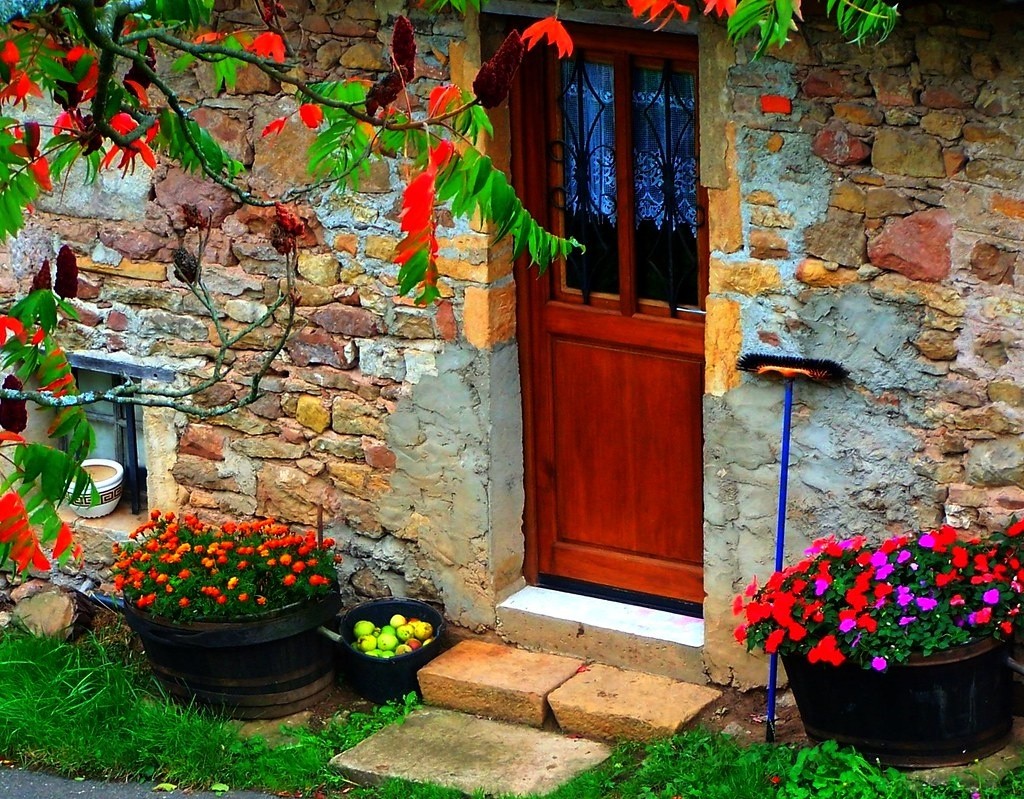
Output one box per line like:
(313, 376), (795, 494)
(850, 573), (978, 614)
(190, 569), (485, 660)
(732, 353), (851, 742)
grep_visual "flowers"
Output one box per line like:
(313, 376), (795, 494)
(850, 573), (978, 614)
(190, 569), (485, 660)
(111, 508), (343, 626)
(733, 518), (1024, 669)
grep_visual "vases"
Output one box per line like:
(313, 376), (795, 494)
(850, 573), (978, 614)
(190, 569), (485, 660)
(63, 458), (125, 519)
(122, 577), (341, 720)
(776, 632), (1015, 770)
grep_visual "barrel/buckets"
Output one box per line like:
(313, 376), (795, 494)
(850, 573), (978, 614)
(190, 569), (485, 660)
(339, 596), (444, 706)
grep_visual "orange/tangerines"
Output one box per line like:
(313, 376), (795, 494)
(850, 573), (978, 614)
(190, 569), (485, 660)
(350, 614), (433, 659)
